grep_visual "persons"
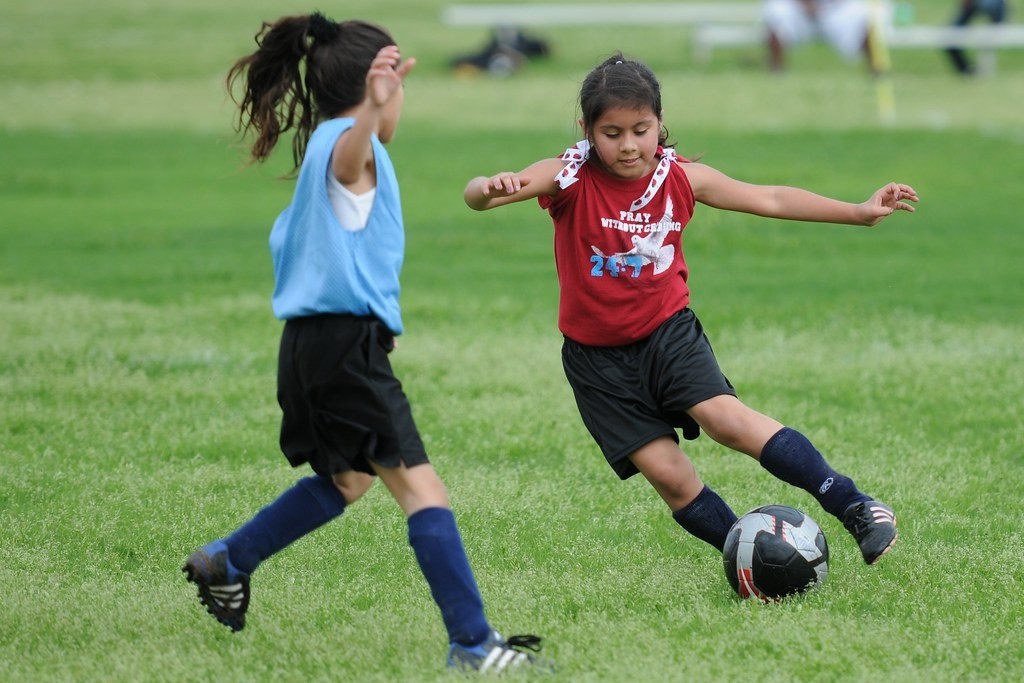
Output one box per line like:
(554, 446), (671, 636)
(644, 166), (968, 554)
(763, 0), (871, 77)
(180, 11), (557, 674)
(464, 55), (918, 564)
(947, 0), (1005, 77)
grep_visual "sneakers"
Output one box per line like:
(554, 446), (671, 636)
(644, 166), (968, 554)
(445, 627), (559, 678)
(842, 500), (899, 566)
(181, 540), (251, 634)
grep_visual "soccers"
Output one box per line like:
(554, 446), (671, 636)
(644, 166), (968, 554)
(721, 504), (831, 602)
